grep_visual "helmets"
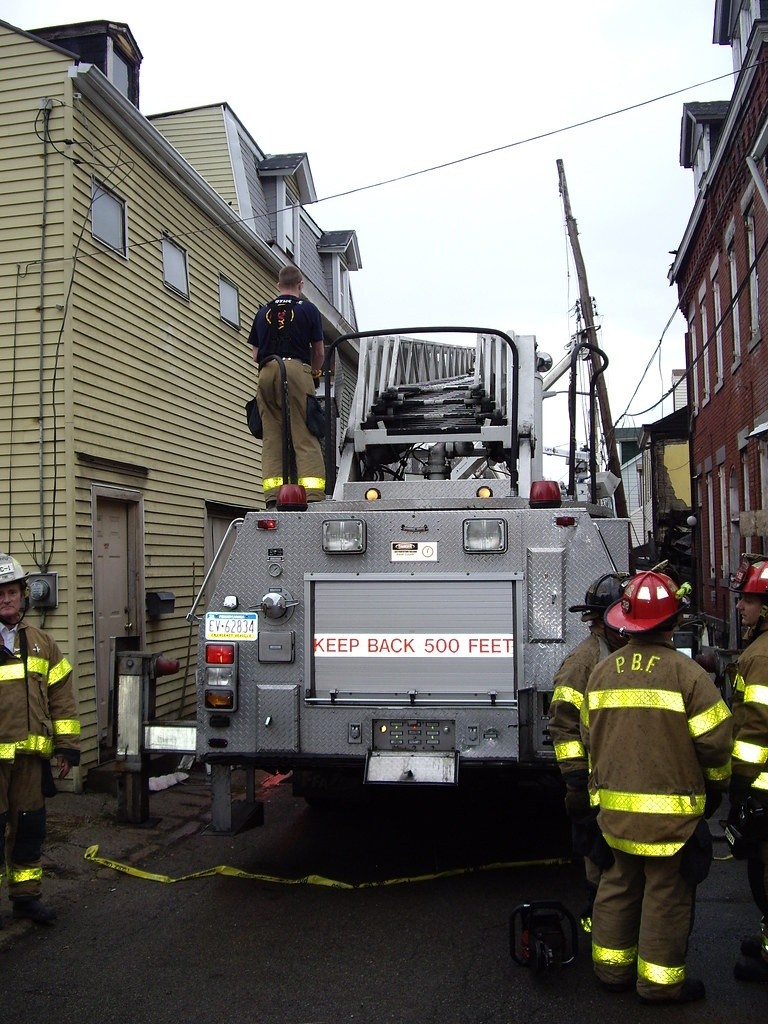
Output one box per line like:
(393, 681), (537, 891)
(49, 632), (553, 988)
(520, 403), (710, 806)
(569, 572), (629, 612)
(602, 571), (691, 633)
(0, 554), (27, 584)
(729, 559), (768, 593)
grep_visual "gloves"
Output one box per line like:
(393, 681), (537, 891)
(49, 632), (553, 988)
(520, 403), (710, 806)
(314, 377), (320, 389)
(727, 773), (755, 817)
(563, 770), (599, 820)
(703, 776), (728, 820)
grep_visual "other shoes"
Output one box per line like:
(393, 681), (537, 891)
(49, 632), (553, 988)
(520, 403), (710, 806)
(265, 499), (277, 509)
(600, 980), (637, 992)
(638, 979), (706, 1004)
(265, 500), (278, 509)
(307, 494), (325, 503)
(13, 896), (57, 920)
(734, 957), (768, 984)
(740, 936), (764, 959)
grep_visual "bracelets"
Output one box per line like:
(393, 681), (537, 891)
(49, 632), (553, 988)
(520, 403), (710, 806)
(312, 369), (322, 379)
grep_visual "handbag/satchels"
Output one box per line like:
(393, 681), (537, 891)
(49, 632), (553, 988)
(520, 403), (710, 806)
(245, 397), (263, 441)
(684, 820), (714, 886)
(720, 794), (765, 859)
(305, 393), (325, 439)
(570, 816), (615, 871)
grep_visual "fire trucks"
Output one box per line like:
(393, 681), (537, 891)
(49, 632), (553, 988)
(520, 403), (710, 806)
(190, 322), (634, 855)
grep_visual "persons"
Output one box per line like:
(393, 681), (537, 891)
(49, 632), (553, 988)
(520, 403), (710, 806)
(0, 553), (82, 930)
(248, 265), (325, 510)
(547, 559), (768, 1003)
